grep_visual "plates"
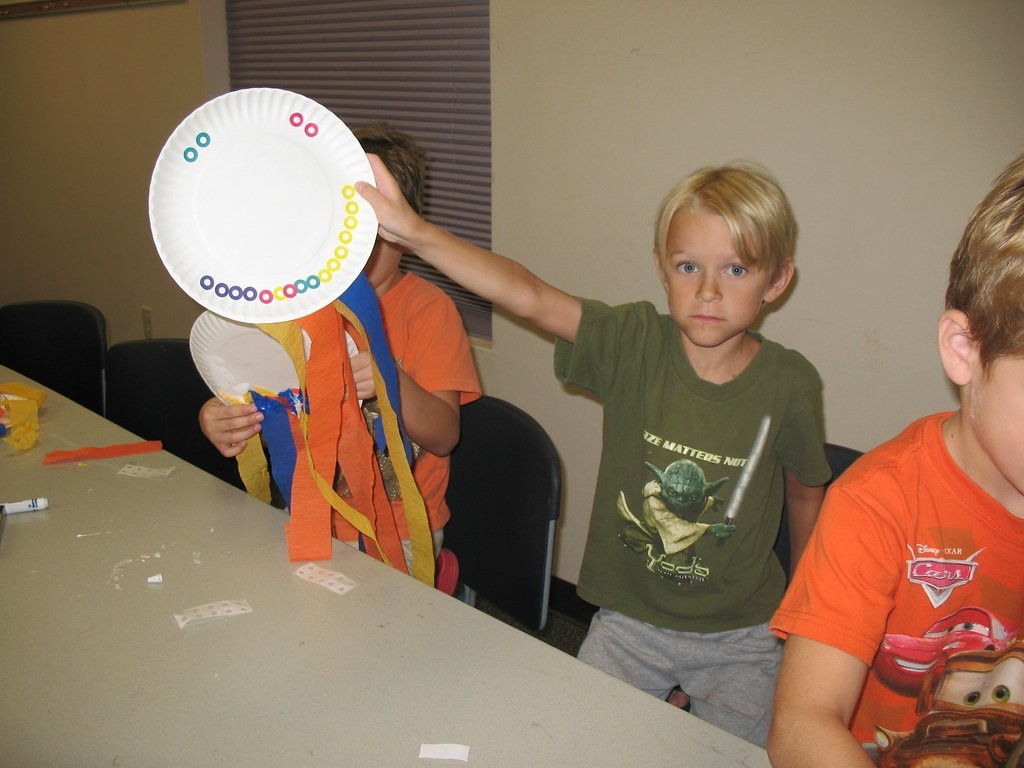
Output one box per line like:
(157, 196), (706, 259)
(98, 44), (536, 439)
(144, 86), (379, 328)
(189, 310), (362, 410)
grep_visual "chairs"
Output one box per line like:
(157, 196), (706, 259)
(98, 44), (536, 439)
(0, 301), (248, 492)
(443, 395), (563, 633)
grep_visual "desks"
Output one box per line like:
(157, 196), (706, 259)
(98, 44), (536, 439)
(0, 362), (774, 767)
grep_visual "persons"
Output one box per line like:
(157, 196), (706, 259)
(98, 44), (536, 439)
(198, 123), (481, 592)
(356, 152), (833, 752)
(764, 151), (1024, 768)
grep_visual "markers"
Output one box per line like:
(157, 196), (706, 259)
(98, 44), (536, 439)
(0, 497), (49, 517)
